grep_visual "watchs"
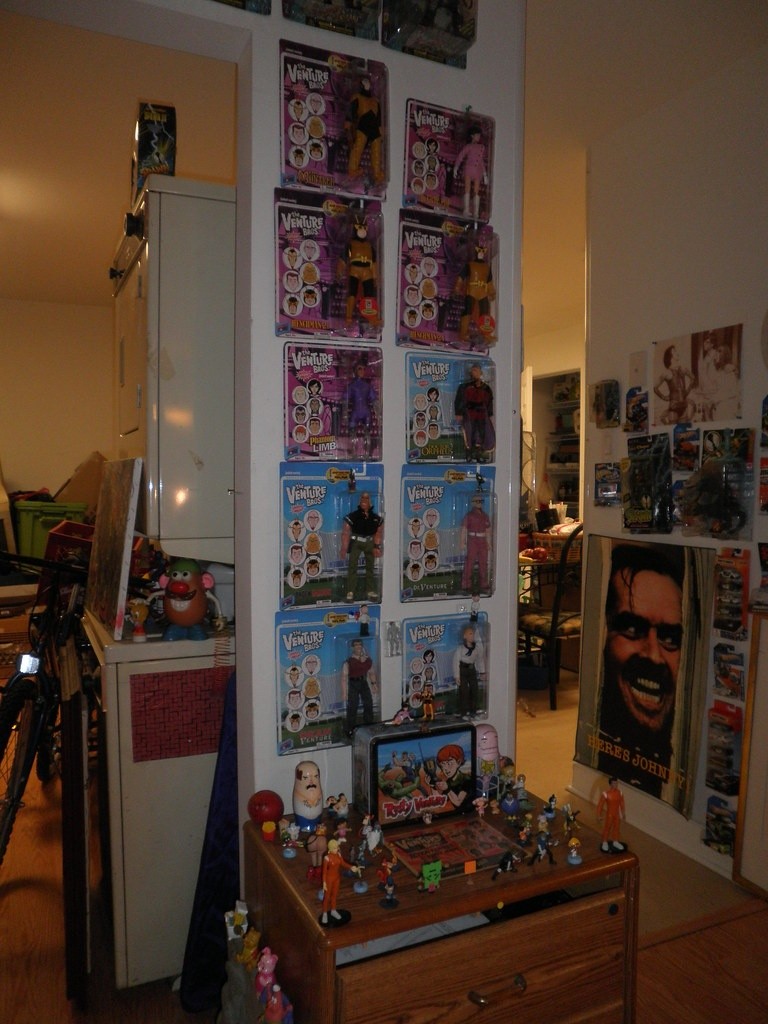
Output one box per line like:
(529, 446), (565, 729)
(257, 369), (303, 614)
(374, 544), (381, 549)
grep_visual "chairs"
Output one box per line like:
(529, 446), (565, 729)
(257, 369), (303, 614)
(519, 523), (583, 711)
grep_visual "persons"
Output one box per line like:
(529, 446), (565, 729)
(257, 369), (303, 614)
(336, 362), (375, 453)
(460, 496), (492, 592)
(335, 223), (383, 327)
(453, 128), (489, 219)
(453, 626), (486, 722)
(344, 75), (384, 181)
(293, 761), (399, 926)
(599, 546), (695, 796)
(454, 364), (493, 463)
(341, 639), (378, 739)
(473, 724), (626, 880)
(340, 492), (384, 602)
(653, 334), (737, 423)
(453, 246), (495, 345)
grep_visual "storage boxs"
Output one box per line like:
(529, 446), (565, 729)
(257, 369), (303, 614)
(131, 97), (176, 208)
(15, 501), (94, 570)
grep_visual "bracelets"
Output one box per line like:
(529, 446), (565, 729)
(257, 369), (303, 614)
(372, 682), (376, 684)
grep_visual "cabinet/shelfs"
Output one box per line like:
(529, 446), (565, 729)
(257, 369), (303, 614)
(108, 173), (237, 564)
(243, 785), (639, 1024)
(547, 402), (580, 508)
(54, 611), (236, 997)
